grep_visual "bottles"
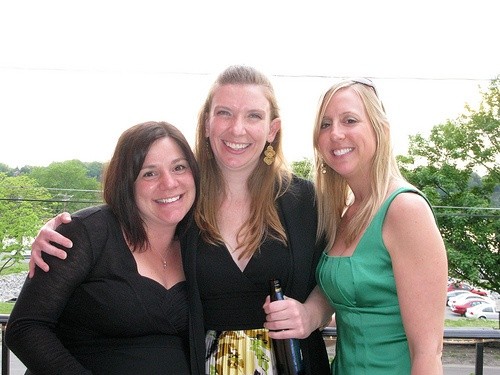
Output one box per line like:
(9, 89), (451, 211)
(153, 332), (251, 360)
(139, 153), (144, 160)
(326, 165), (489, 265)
(268, 279), (306, 375)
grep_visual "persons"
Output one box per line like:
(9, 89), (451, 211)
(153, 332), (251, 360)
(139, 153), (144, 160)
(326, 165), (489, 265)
(4, 120), (206, 375)
(312, 76), (448, 375)
(30, 64), (336, 375)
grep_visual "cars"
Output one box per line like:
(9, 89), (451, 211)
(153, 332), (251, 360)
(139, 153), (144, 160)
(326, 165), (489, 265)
(445, 277), (499, 320)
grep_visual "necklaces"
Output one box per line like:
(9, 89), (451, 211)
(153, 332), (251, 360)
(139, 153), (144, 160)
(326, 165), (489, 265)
(160, 255), (167, 267)
